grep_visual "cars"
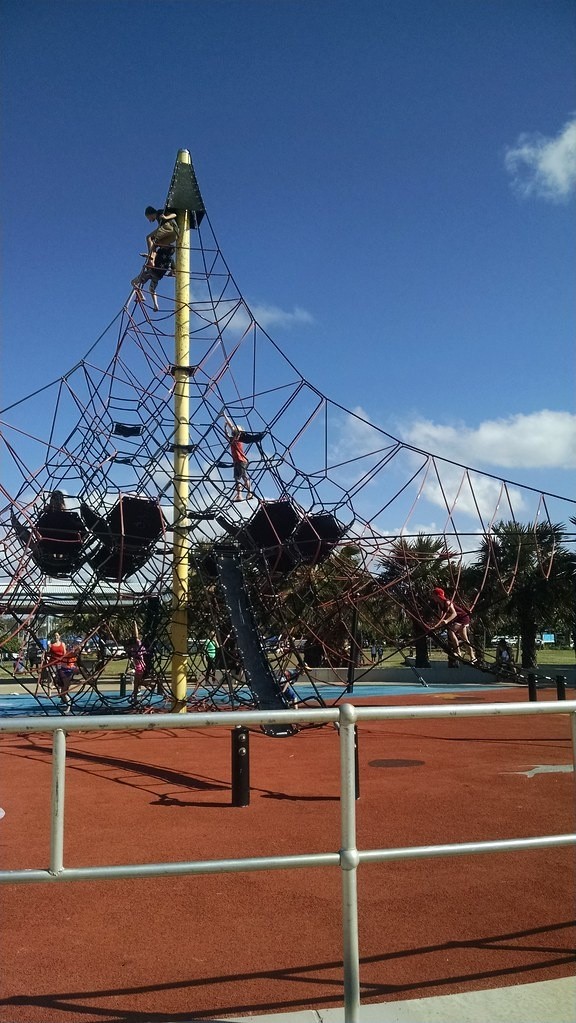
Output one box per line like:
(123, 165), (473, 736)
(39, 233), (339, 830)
(433, 629), (544, 650)
(17, 637), (105, 664)
(265, 642), (289, 653)
(106, 644), (126, 656)
(562, 639), (575, 649)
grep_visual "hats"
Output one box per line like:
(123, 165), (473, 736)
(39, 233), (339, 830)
(433, 587), (446, 599)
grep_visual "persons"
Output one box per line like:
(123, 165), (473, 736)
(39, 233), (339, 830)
(376, 644), (383, 663)
(27, 642), (39, 674)
(541, 644), (544, 649)
(476, 638), (518, 677)
(370, 642), (377, 663)
(222, 407), (253, 502)
(33, 489), (89, 581)
(144, 205), (179, 270)
(13, 657), (27, 676)
(17, 648), (24, 659)
(56, 643), (82, 704)
(41, 631), (67, 699)
(122, 620), (154, 699)
(202, 630), (220, 686)
(276, 660), (312, 710)
(432, 586), (478, 665)
(131, 243), (175, 312)
